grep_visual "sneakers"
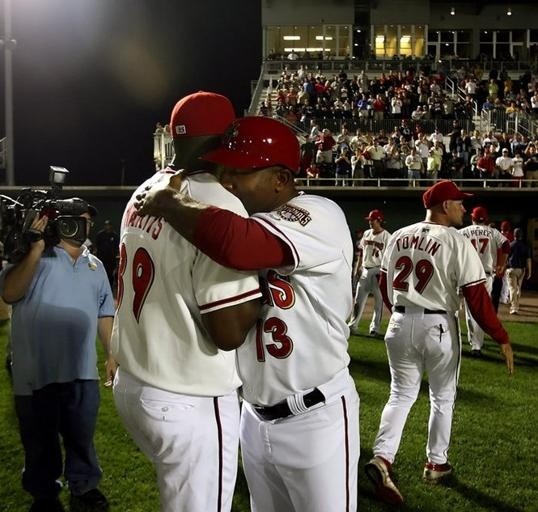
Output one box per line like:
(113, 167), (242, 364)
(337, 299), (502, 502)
(423, 463), (452, 479)
(365, 456), (403, 504)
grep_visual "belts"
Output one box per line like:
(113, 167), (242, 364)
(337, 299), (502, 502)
(253, 391), (323, 420)
(394, 306), (446, 314)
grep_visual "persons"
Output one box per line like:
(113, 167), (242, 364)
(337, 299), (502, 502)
(456, 207), (511, 355)
(260, 50), (537, 187)
(489, 220), (531, 314)
(1, 198), (116, 512)
(365, 179), (514, 505)
(110, 91), (263, 511)
(348, 210), (391, 336)
(95, 220), (120, 286)
(133, 117), (360, 512)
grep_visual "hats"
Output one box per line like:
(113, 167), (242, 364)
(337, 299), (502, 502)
(470, 207), (487, 218)
(169, 92), (300, 172)
(422, 181), (473, 209)
(365, 210), (384, 221)
